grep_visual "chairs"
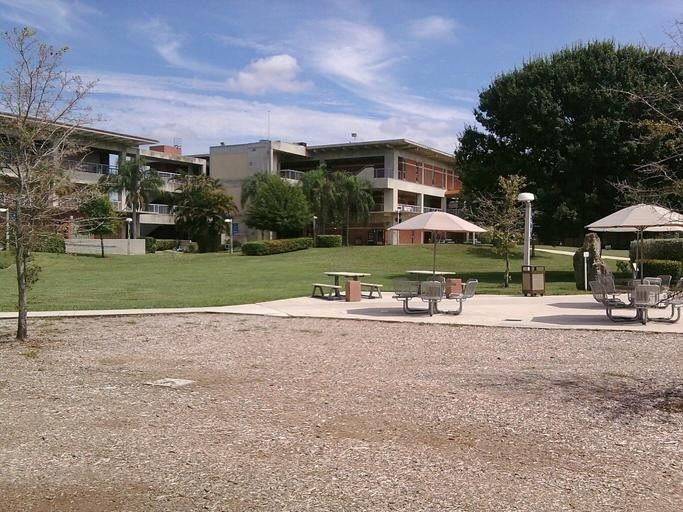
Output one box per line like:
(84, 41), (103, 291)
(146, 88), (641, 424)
(392, 278), (478, 317)
(588, 274), (683, 325)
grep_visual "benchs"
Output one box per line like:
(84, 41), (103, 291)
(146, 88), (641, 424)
(358, 281), (383, 299)
(310, 281), (342, 300)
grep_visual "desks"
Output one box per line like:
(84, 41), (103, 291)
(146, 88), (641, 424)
(325, 271), (370, 299)
(406, 270), (456, 295)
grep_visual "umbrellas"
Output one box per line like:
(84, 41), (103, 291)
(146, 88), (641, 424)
(583, 203), (683, 287)
(386, 210), (487, 282)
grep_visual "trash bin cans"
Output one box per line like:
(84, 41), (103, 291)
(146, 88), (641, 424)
(521, 265), (545, 297)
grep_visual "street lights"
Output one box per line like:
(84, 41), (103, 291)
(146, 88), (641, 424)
(0, 208), (9, 251)
(125, 217), (132, 256)
(223, 219), (232, 254)
(395, 209), (400, 246)
(312, 216), (317, 248)
(517, 193), (534, 272)
